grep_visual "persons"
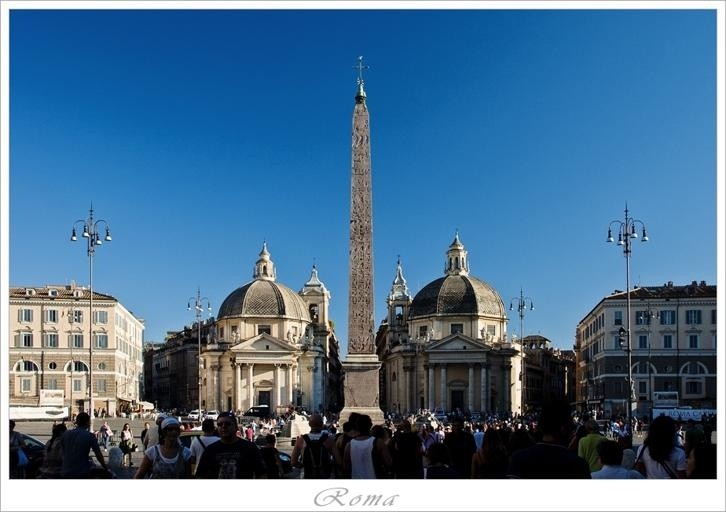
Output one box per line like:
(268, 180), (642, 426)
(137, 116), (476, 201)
(9, 405), (716, 480)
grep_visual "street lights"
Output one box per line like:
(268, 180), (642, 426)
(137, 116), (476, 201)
(510, 286), (534, 420)
(606, 199), (649, 446)
(69, 198), (112, 433)
(640, 305), (661, 408)
(186, 284), (211, 420)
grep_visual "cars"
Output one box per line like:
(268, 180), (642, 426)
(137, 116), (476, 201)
(16, 433), (46, 465)
(177, 430), (292, 473)
(187, 409), (220, 421)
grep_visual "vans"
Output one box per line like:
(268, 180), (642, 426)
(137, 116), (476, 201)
(242, 405), (270, 416)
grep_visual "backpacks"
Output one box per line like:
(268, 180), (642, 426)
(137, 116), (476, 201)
(42, 435), (65, 473)
(302, 432), (331, 479)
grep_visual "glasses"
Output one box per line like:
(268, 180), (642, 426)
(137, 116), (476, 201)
(217, 422), (234, 426)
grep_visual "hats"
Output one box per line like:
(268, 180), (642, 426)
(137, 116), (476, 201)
(685, 428), (705, 441)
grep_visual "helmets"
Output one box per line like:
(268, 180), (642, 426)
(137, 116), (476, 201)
(160, 417), (181, 431)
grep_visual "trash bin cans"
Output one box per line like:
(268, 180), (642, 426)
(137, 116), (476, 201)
(109, 447), (122, 469)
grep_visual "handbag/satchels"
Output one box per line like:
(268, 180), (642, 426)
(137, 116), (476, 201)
(631, 444), (648, 479)
